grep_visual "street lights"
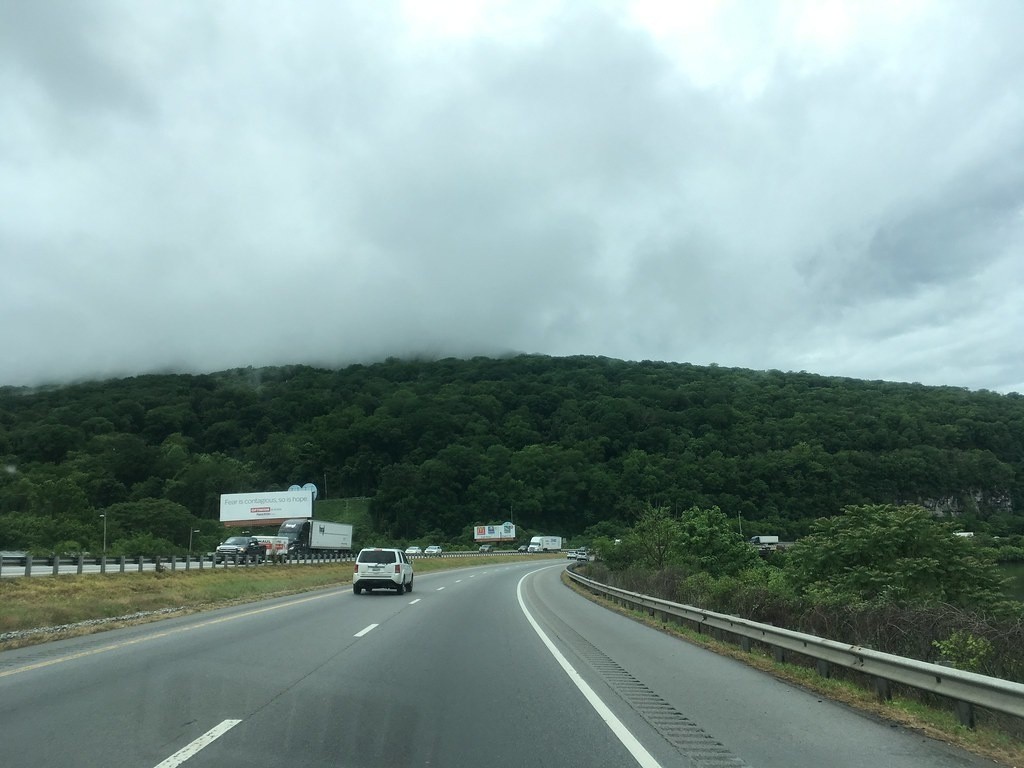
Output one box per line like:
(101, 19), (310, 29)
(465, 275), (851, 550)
(100, 512), (106, 551)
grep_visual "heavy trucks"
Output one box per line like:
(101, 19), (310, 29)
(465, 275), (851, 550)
(277, 519), (353, 559)
(750, 536), (778, 545)
(528, 536), (562, 553)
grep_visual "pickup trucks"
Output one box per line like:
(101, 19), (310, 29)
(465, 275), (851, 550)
(215, 537), (266, 565)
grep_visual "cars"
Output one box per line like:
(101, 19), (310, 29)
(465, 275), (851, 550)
(352, 548), (414, 595)
(576, 552), (590, 561)
(567, 551), (578, 559)
(479, 545), (493, 553)
(405, 546), (422, 556)
(424, 546), (442, 556)
(518, 545), (528, 553)
